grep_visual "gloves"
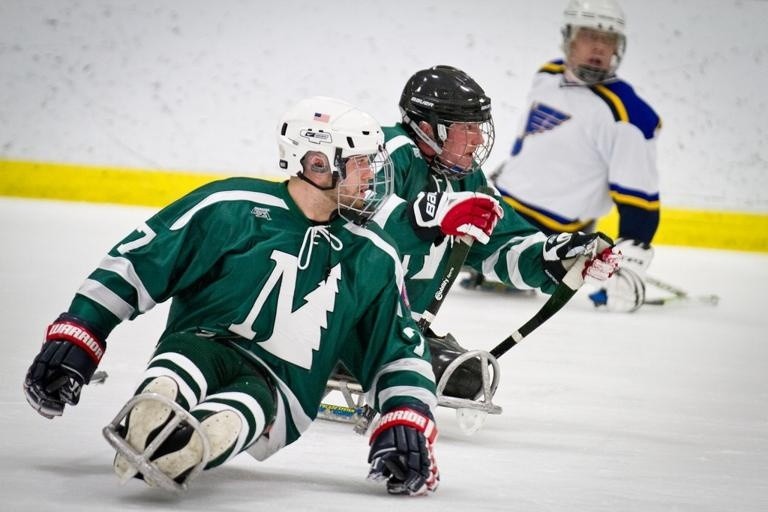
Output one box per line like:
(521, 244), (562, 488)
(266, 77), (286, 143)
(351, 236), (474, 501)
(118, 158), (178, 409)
(543, 228), (623, 282)
(24, 312), (108, 420)
(366, 404), (440, 496)
(408, 190), (506, 248)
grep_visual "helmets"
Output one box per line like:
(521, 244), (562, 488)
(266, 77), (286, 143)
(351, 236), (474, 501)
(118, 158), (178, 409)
(558, 0), (630, 83)
(275, 96), (397, 230)
(397, 63), (496, 183)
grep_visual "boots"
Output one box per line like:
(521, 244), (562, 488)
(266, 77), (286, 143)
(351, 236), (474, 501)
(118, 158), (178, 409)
(147, 409), (243, 486)
(112, 374), (178, 479)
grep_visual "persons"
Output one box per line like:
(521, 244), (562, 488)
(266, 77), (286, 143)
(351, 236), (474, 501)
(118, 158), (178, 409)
(22, 95), (441, 497)
(330, 64), (625, 438)
(460, 0), (665, 315)
